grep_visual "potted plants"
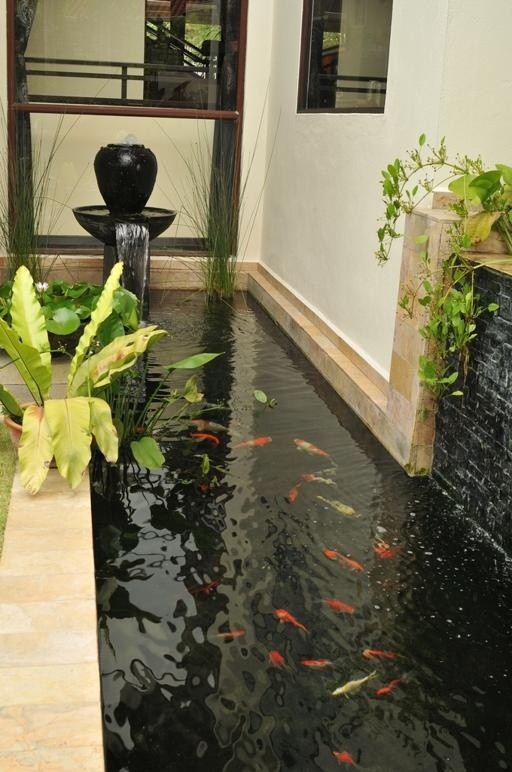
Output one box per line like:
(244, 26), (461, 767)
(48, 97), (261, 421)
(0, 263), (171, 494)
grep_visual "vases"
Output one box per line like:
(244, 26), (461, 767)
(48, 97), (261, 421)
(93, 143), (158, 214)
